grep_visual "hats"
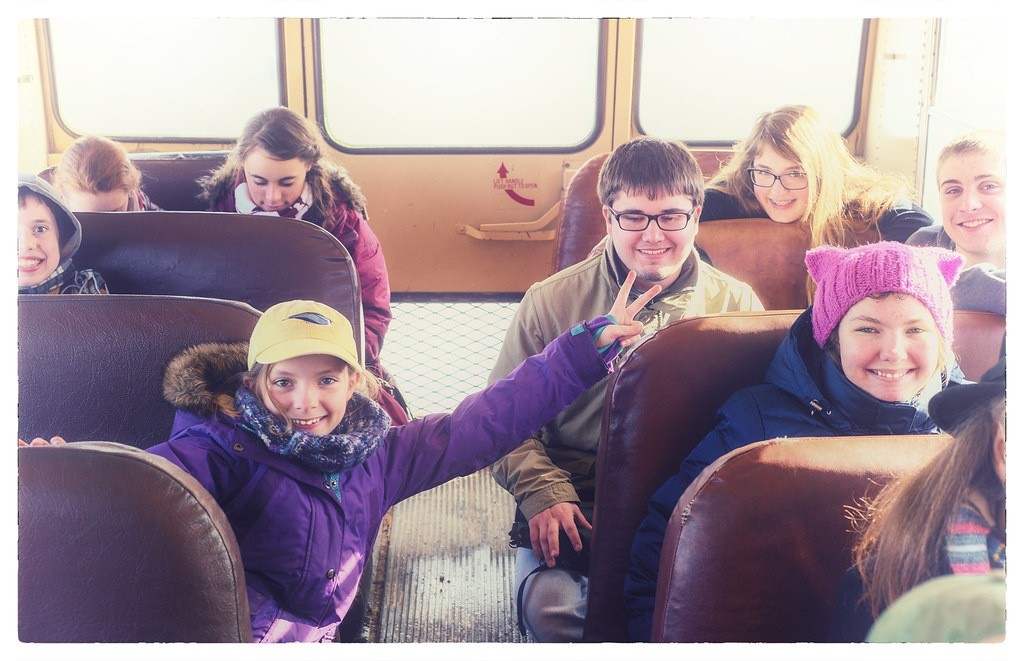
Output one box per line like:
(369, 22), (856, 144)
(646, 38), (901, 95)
(247, 300), (363, 376)
(927, 330), (1005, 434)
(804, 242), (964, 347)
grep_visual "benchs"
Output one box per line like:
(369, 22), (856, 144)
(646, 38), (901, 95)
(19, 148), (366, 643)
(553, 147), (1006, 644)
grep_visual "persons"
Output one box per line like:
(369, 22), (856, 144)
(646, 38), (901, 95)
(18, 269), (664, 644)
(19, 172), (112, 296)
(490, 136), (766, 644)
(857, 127), (1007, 642)
(199, 105), (412, 427)
(57, 137), (169, 211)
(697, 101), (934, 243)
(620, 239), (973, 642)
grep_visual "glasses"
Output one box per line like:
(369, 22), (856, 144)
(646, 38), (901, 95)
(610, 206), (696, 231)
(747, 161), (808, 190)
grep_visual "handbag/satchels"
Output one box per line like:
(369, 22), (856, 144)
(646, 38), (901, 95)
(508, 374), (614, 566)
